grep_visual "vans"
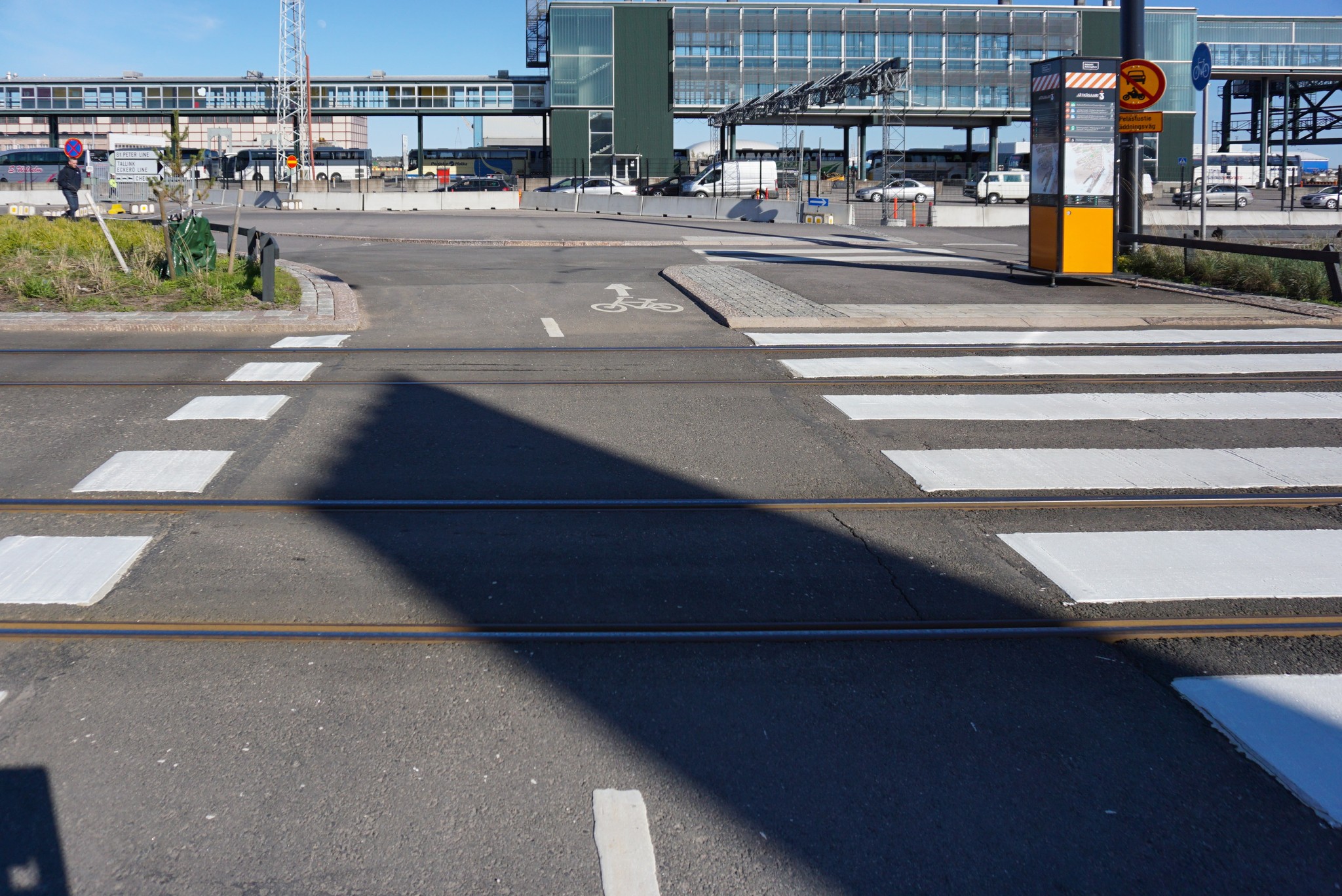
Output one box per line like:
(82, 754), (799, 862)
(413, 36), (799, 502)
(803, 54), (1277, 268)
(963, 171), (1030, 204)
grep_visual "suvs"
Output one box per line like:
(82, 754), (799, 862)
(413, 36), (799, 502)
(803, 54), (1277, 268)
(430, 179), (512, 192)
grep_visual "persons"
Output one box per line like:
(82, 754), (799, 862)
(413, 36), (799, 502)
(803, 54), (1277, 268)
(57, 157), (82, 222)
(109, 175), (119, 200)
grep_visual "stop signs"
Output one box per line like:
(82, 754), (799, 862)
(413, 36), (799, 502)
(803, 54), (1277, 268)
(287, 155), (298, 169)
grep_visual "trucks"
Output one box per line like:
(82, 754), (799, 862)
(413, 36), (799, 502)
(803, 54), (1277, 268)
(678, 159), (780, 200)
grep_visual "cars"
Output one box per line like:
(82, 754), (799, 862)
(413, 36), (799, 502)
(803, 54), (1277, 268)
(1300, 184), (1342, 209)
(1171, 183), (1255, 208)
(532, 177), (593, 192)
(855, 178), (935, 204)
(549, 178), (638, 196)
(640, 175), (704, 196)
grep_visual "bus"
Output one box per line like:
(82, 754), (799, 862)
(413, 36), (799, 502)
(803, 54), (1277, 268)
(0, 147), (221, 181)
(1192, 152), (1302, 189)
(866, 148), (990, 184)
(401, 146), (532, 179)
(224, 142), (373, 182)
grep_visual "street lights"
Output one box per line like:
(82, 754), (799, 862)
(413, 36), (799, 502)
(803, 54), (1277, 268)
(1298, 148), (1309, 174)
(776, 142), (782, 148)
